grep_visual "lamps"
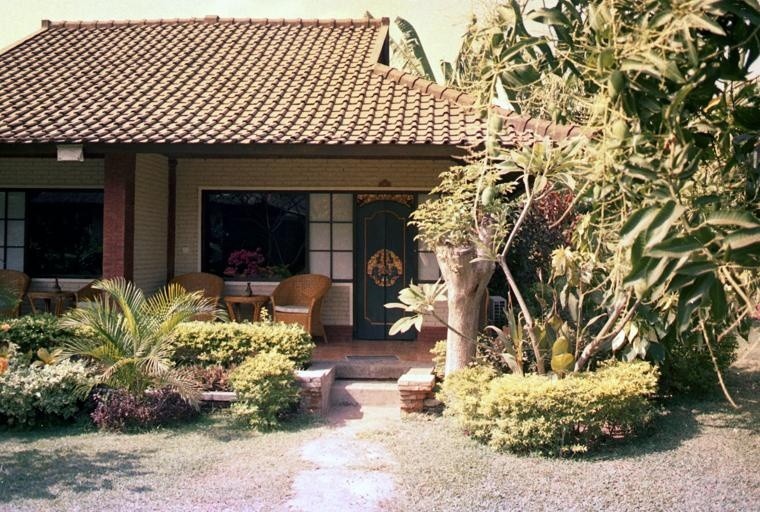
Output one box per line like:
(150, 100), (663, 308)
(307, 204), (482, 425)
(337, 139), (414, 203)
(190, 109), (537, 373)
(54, 144), (85, 163)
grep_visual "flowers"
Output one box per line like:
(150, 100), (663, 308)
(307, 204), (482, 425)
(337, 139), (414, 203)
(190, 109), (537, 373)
(222, 248), (265, 284)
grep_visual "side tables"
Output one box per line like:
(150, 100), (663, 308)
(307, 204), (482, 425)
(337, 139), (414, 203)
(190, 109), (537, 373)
(26, 291), (78, 317)
(224, 293), (271, 323)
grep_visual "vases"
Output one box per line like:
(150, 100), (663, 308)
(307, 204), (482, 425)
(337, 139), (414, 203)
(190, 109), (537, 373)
(243, 281), (253, 297)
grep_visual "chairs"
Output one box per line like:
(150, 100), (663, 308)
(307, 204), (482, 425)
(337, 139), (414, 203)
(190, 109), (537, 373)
(167, 271), (225, 323)
(0, 269), (31, 319)
(269, 272), (332, 345)
